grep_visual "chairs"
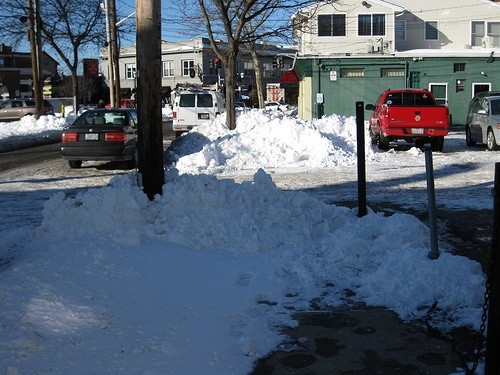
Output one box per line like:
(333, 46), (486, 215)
(93, 117), (105, 124)
(113, 118), (124, 124)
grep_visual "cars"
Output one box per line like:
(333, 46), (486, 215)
(465, 91), (500, 151)
(0, 97), (55, 122)
(104, 99), (136, 108)
(60, 108), (137, 168)
(251, 101), (289, 112)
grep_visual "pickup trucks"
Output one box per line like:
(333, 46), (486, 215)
(365, 89), (450, 152)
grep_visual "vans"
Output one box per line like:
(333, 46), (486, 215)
(172, 90), (226, 137)
